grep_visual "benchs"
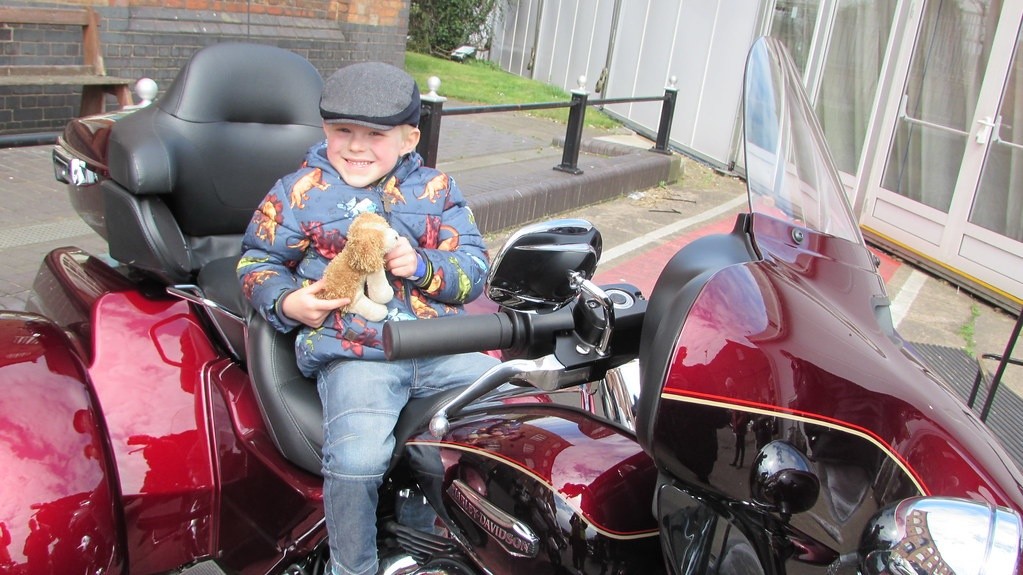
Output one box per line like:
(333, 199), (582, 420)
(0, 6), (134, 146)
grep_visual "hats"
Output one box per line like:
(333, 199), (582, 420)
(319, 61), (422, 130)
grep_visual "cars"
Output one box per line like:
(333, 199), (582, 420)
(450, 44), (477, 63)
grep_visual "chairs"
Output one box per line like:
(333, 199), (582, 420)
(103, 42), (321, 284)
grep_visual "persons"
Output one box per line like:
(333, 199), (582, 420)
(234, 61), (536, 575)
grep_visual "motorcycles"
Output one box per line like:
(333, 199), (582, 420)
(0, 33), (1022, 574)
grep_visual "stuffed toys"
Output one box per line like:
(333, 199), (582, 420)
(312, 211), (397, 323)
